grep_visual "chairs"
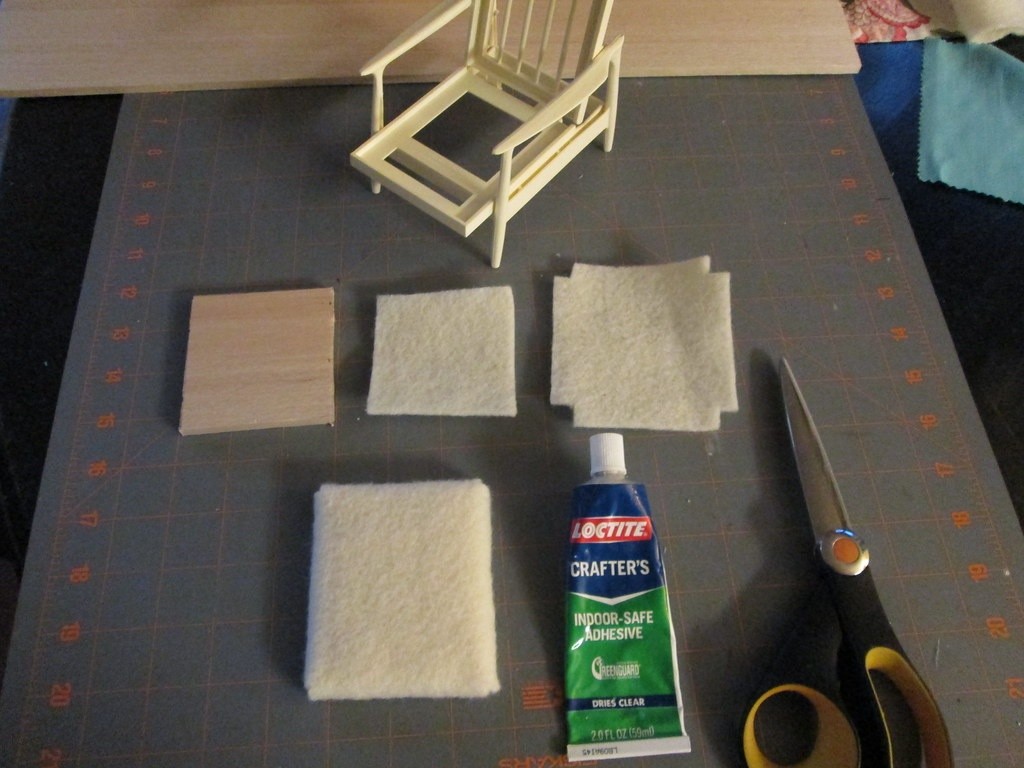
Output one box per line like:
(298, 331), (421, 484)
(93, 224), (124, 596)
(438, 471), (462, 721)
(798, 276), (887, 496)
(359, 0), (624, 269)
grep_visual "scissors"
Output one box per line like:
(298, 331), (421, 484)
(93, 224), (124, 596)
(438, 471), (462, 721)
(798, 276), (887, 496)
(740, 356), (954, 768)
